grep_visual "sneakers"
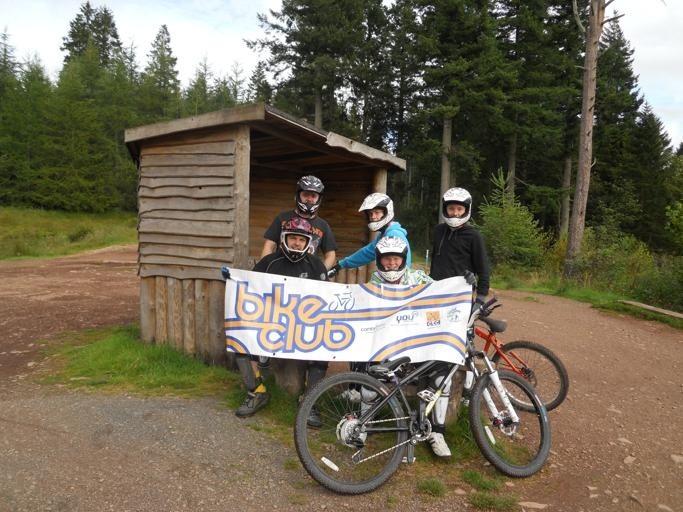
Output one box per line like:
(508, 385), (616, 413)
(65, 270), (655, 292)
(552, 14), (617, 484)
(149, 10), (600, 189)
(297, 397), (321, 428)
(334, 389), (360, 401)
(425, 429), (452, 458)
(310, 404), (321, 416)
(234, 390), (271, 418)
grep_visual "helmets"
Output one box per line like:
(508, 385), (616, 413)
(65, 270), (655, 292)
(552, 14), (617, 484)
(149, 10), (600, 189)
(278, 216), (314, 263)
(374, 236), (408, 282)
(357, 192), (395, 233)
(293, 174), (326, 215)
(442, 186), (472, 228)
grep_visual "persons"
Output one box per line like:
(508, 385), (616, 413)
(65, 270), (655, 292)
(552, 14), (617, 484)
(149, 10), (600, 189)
(328, 191), (413, 403)
(358, 233), (479, 458)
(259, 173), (336, 273)
(426, 184), (492, 408)
(218, 215), (331, 429)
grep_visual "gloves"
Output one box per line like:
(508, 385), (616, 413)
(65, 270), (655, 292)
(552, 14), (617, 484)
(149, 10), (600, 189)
(326, 260), (342, 279)
(219, 265), (230, 281)
(461, 268), (479, 288)
(470, 294), (486, 315)
